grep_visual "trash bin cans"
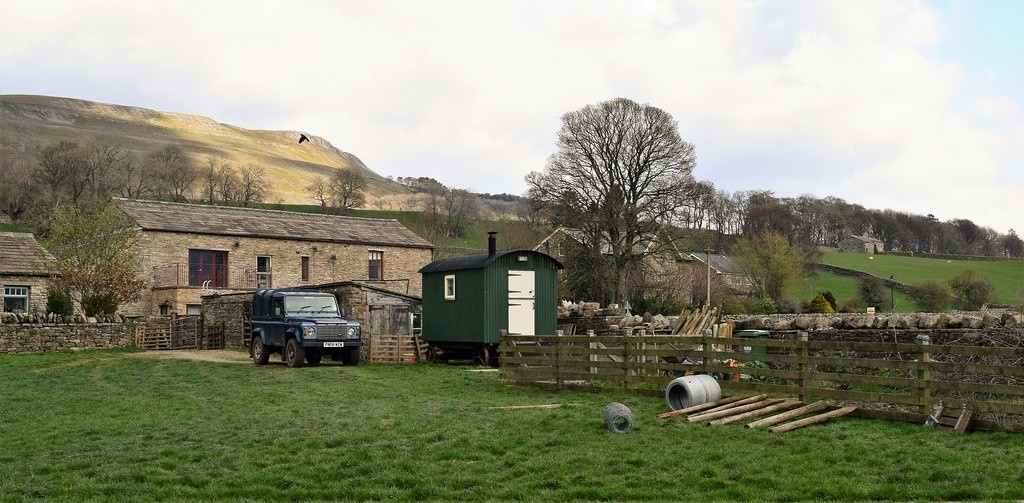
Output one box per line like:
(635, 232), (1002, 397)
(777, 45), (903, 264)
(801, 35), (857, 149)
(736, 329), (770, 381)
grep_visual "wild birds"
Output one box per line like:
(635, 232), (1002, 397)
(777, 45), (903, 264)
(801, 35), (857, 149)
(299, 134), (310, 144)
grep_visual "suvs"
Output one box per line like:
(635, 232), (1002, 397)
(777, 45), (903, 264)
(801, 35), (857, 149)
(248, 288), (363, 368)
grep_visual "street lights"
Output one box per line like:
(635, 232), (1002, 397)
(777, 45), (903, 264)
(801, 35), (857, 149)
(889, 274), (895, 314)
